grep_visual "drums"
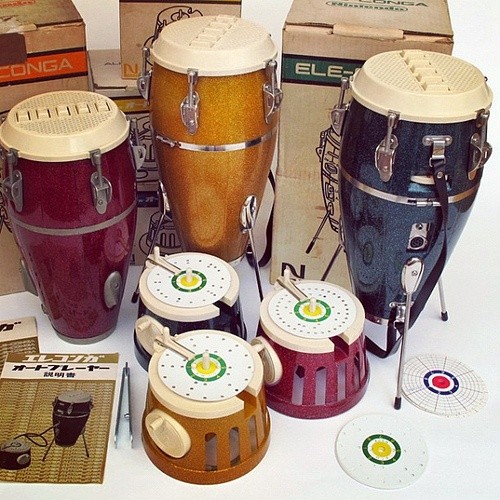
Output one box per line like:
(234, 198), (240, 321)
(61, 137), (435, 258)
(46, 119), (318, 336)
(334, 48), (495, 325)
(256, 269), (371, 419)
(137, 14), (284, 269)
(133, 245), (245, 375)
(0, 442), (34, 470)
(51, 390), (94, 449)
(140, 324), (270, 485)
(0, 88), (144, 346)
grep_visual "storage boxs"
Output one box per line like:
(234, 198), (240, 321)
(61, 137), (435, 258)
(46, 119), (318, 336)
(269, 0), (455, 297)
(0, 0), (243, 297)
